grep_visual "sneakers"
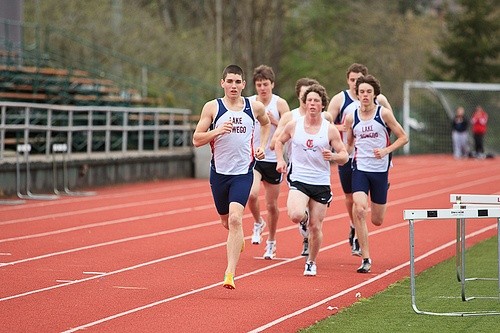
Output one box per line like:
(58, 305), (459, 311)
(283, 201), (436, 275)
(348, 225), (357, 246)
(352, 239), (363, 257)
(300, 239), (310, 257)
(222, 271), (236, 290)
(303, 261), (318, 276)
(264, 239), (279, 261)
(240, 227), (246, 253)
(251, 217), (266, 245)
(298, 209), (309, 239)
(357, 258), (374, 272)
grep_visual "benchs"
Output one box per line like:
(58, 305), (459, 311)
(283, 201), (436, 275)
(0, 48), (200, 147)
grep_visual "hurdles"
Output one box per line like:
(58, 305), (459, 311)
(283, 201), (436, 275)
(403, 194), (500, 317)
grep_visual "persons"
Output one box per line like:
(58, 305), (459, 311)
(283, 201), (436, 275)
(450, 104), (491, 161)
(244, 65), (291, 259)
(192, 65), (271, 289)
(270, 62), (409, 276)
